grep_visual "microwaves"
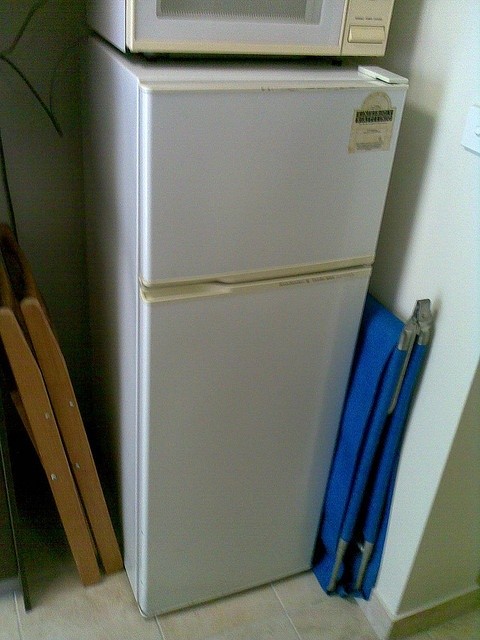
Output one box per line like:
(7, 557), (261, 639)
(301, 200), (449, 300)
(88, 0), (396, 67)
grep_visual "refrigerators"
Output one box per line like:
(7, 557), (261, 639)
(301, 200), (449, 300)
(80, 31), (408, 621)
